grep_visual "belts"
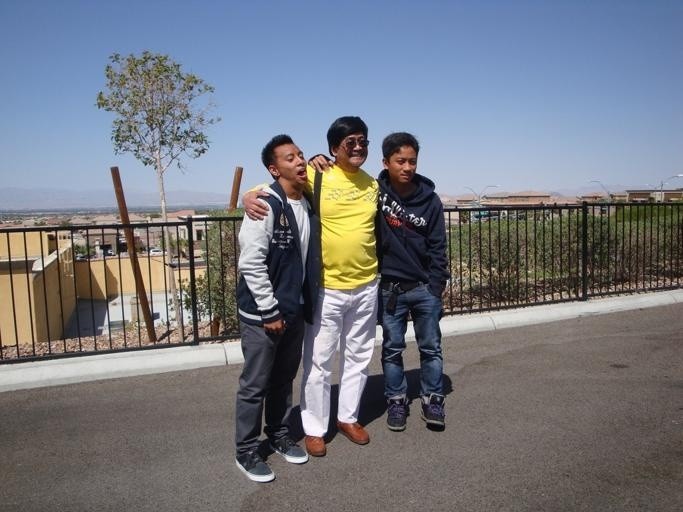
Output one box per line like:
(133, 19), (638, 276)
(379, 279), (428, 315)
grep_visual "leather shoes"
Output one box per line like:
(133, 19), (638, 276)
(306, 435), (326, 457)
(334, 418), (370, 444)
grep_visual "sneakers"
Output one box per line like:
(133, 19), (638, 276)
(419, 392), (446, 426)
(236, 450), (276, 482)
(386, 397), (409, 431)
(268, 435), (308, 464)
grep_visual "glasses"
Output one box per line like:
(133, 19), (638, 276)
(340, 140), (370, 148)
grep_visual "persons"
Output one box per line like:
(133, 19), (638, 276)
(240, 115), (380, 456)
(307, 131), (451, 432)
(235, 135), (322, 484)
